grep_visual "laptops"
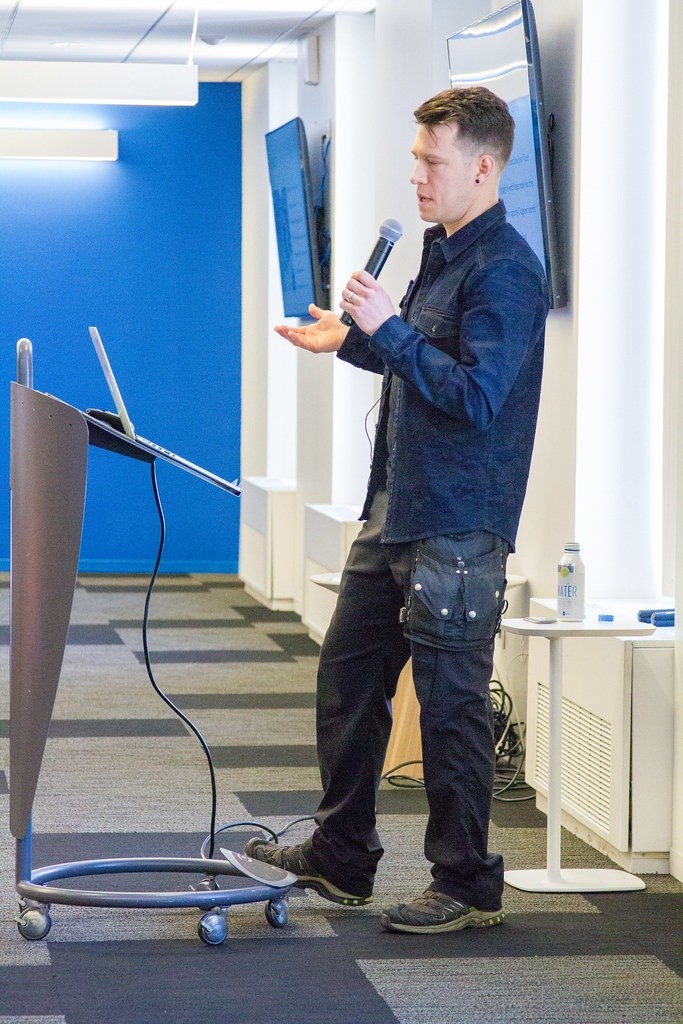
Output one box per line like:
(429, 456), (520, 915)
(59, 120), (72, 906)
(89, 327), (242, 499)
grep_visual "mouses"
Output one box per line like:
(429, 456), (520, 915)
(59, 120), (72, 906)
(86, 408), (135, 434)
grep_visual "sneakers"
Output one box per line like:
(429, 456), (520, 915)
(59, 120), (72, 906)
(381, 889), (507, 934)
(243, 836), (375, 906)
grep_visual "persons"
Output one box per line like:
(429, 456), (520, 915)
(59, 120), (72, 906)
(245, 84), (549, 934)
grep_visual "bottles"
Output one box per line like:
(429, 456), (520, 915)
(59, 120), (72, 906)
(557, 541), (585, 623)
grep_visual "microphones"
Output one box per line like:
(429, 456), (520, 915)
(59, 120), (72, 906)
(339, 218), (404, 326)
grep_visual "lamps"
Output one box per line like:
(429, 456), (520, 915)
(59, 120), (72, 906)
(0, 0), (199, 106)
(0, 116), (118, 162)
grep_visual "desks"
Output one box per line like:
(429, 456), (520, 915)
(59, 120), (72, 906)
(498, 617), (657, 893)
(310, 572), (527, 596)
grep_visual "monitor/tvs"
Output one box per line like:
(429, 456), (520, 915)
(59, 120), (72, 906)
(446, 0), (568, 309)
(265, 118), (329, 319)
